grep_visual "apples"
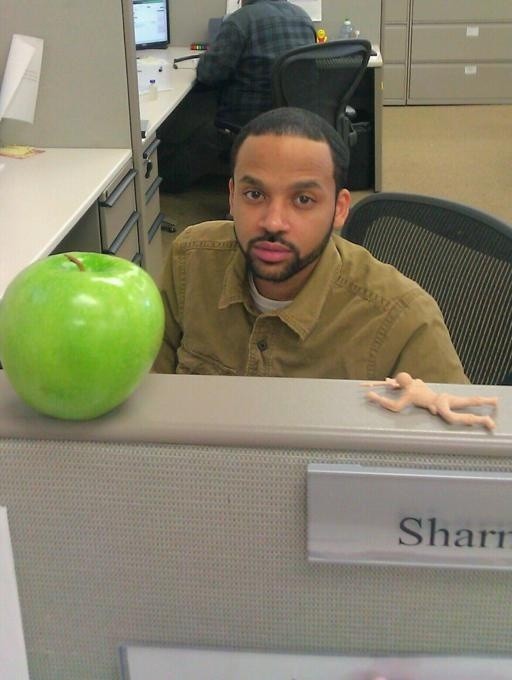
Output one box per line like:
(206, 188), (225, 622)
(0, 251), (166, 421)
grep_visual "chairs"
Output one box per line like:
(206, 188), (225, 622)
(215, 39), (371, 176)
(340, 193), (512, 385)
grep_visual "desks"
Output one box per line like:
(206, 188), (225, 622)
(137, 46), (383, 275)
(0, 147), (142, 295)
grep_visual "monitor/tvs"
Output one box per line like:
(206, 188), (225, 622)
(132, 0), (171, 50)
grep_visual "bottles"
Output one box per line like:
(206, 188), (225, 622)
(340, 18), (353, 40)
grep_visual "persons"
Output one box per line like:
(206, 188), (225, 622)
(156, 0), (318, 195)
(148, 106), (471, 385)
